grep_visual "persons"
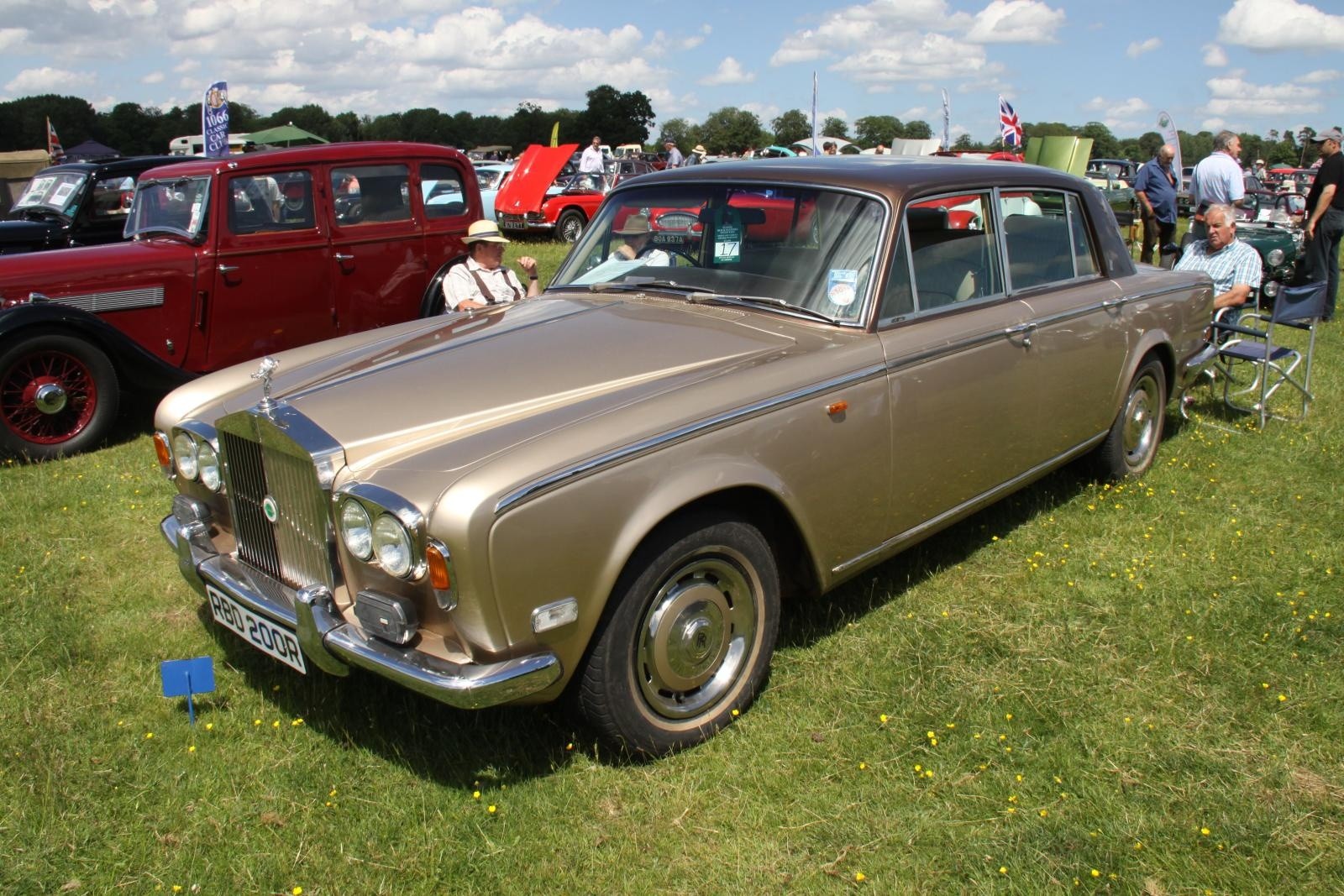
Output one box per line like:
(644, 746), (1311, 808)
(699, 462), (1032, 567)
(936, 146), (943, 152)
(659, 137), (684, 170)
(461, 145), (581, 190)
(716, 136), (892, 160)
(684, 144), (707, 167)
(578, 136), (607, 191)
(1292, 128), (1344, 323)
(607, 213), (670, 268)
(90, 145), (362, 226)
(1235, 155), (1324, 213)
(1171, 203), (1263, 348)
(441, 219), (539, 314)
(1086, 152), (1131, 177)
(1187, 129), (1246, 242)
(1133, 143), (1179, 264)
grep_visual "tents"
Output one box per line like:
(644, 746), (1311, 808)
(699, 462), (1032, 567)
(62, 139), (122, 162)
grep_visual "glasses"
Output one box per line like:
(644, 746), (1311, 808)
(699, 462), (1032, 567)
(1319, 140), (1332, 144)
(622, 235), (646, 239)
(478, 241), (503, 248)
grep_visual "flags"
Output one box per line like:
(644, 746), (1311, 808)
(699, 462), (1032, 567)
(1000, 94), (1023, 151)
(49, 120), (69, 165)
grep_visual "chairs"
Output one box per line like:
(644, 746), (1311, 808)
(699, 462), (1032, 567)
(1208, 280), (1327, 434)
(788, 190), (908, 319)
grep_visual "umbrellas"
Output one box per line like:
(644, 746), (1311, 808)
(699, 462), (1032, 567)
(236, 120), (331, 148)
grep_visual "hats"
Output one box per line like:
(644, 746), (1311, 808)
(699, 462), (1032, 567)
(612, 214), (662, 236)
(691, 145), (707, 154)
(460, 219), (510, 245)
(1310, 128), (1343, 143)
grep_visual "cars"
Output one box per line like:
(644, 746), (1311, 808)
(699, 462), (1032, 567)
(149, 153), (1224, 763)
(1, 140), (1344, 461)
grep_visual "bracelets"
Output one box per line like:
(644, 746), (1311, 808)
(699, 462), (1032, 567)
(527, 275), (539, 280)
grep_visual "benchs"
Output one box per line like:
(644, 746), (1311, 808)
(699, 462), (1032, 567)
(894, 206), (1067, 314)
